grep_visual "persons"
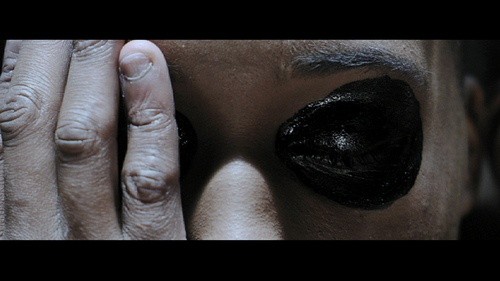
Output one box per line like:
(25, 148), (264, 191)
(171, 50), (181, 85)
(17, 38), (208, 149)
(1, 40), (500, 241)
